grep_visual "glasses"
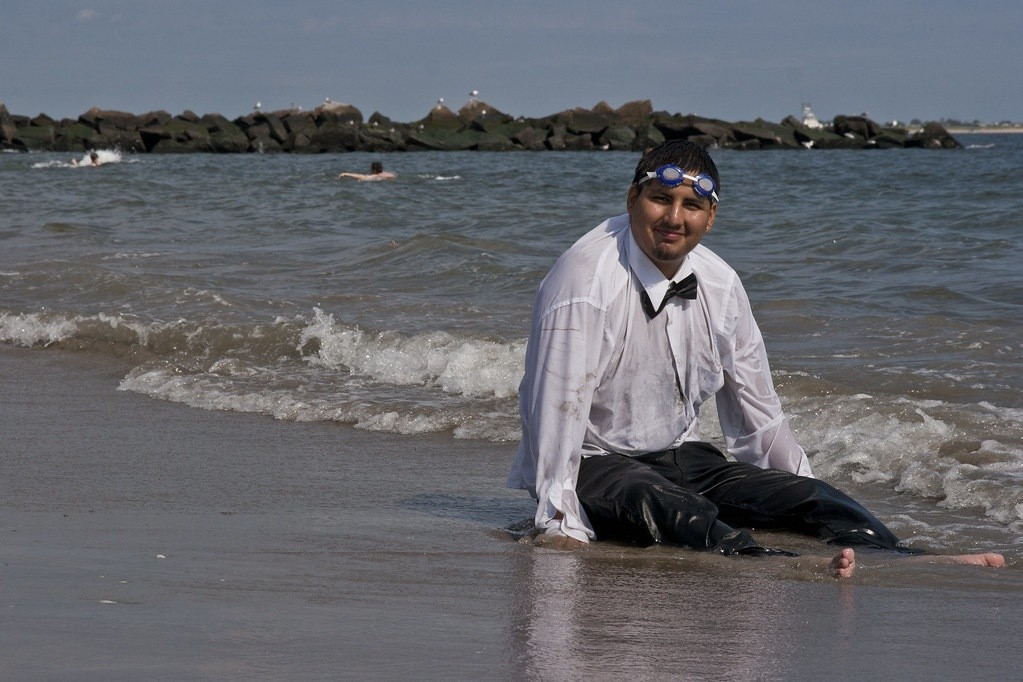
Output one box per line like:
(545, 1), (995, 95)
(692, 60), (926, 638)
(638, 163), (720, 204)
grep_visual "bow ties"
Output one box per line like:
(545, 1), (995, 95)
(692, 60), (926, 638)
(641, 272), (698, 320)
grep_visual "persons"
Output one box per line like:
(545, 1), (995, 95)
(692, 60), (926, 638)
(509, 140), (1005, 579)
(71, 153), (101, 167)
(340, 162), (393, 180)
